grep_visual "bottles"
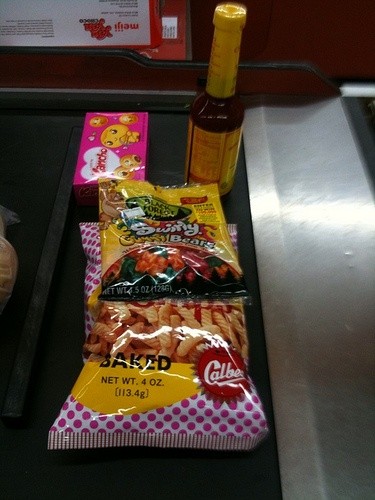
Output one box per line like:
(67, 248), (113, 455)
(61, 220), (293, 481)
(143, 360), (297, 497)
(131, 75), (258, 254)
(184, 3), (248, 200)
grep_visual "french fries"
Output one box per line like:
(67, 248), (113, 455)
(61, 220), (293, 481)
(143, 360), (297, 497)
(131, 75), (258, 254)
(85, 302), (247, 364)
